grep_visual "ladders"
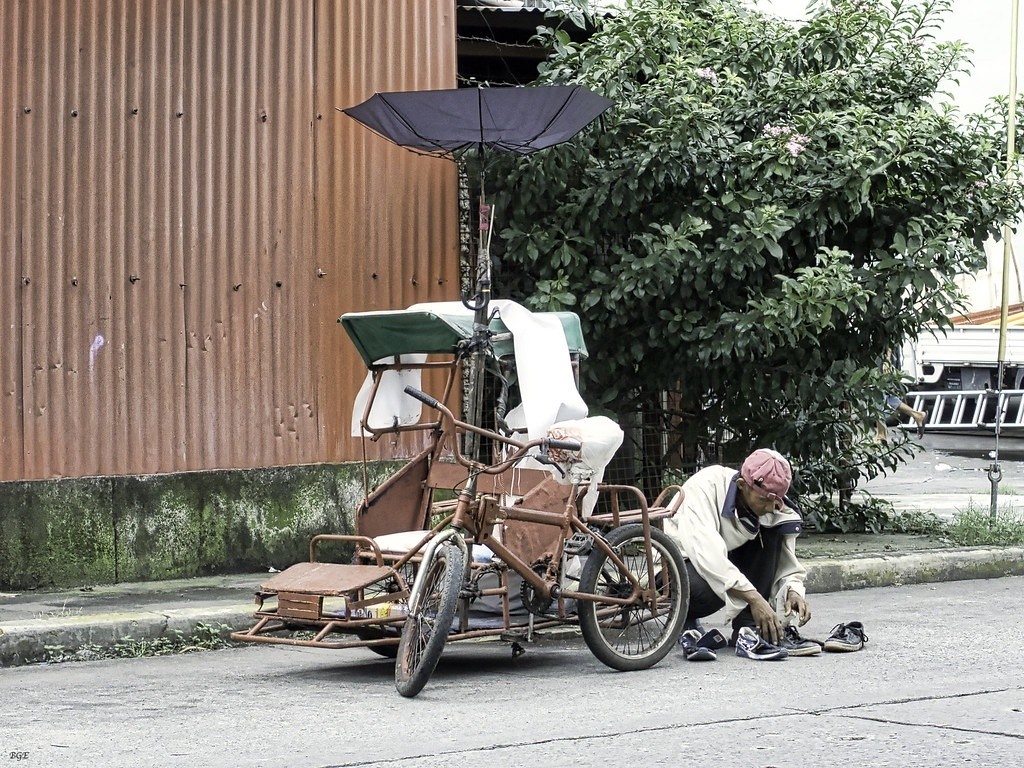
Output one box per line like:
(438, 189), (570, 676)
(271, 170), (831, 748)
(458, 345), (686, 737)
(896, 389), (1024, 429)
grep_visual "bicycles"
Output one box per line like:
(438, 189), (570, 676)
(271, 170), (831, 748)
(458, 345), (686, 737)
(228, 288), (691, 699)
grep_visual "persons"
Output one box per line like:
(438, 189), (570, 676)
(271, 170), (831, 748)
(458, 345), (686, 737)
(874, 395), (928, 441)
(652, 448), (811, 648)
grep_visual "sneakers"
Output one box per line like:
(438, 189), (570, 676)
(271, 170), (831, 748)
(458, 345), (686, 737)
(678, 627), (728, 662)
(734, 627), (788, 662)
(824, 621), (865, 652)
(761, 625), (822, 657)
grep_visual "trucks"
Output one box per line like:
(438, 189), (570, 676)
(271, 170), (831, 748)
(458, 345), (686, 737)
(863, 323), (1024, 431)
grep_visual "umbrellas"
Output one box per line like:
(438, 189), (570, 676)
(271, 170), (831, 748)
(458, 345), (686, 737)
(335, 84), (620, 310)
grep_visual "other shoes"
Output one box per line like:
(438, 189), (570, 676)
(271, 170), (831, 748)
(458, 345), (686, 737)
(682, 617), (707, 635)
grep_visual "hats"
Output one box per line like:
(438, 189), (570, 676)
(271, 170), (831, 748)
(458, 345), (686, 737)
(738, 447), (793, 501)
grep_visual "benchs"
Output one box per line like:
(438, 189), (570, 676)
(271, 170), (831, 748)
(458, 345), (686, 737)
(355, 433), (579, 626)
